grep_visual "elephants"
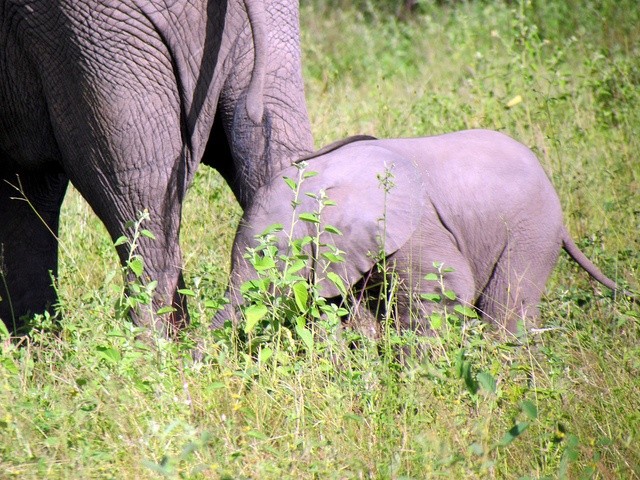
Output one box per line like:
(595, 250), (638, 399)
(0, 1), (317, 363)
(193, 128), (640, 391)
